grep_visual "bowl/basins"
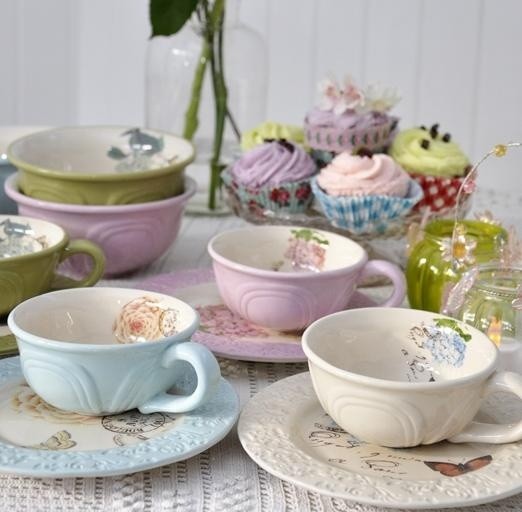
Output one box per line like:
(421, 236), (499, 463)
(9, 125), (195, 206)
(0, 122), (60, 212)
(6, 176), (199, 277)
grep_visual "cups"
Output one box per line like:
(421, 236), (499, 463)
(8, 286), (221, 418)
(301, 305), (522, 449)
(207, 225), (408, 333)
(0, 212), (108, 318)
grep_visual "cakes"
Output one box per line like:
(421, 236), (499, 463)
(219, 139), (318, 214)
(241, 123), (309, 154)
(310, 149), (424, 235)
(302, 77), (399, 164)
(391, 123), (476, 214)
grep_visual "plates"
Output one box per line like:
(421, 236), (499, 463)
(141, 267), (380, 364)
(237, 371), (521, 509)
(0, 354), (239, 477)
(0, 323), (18, 353)
